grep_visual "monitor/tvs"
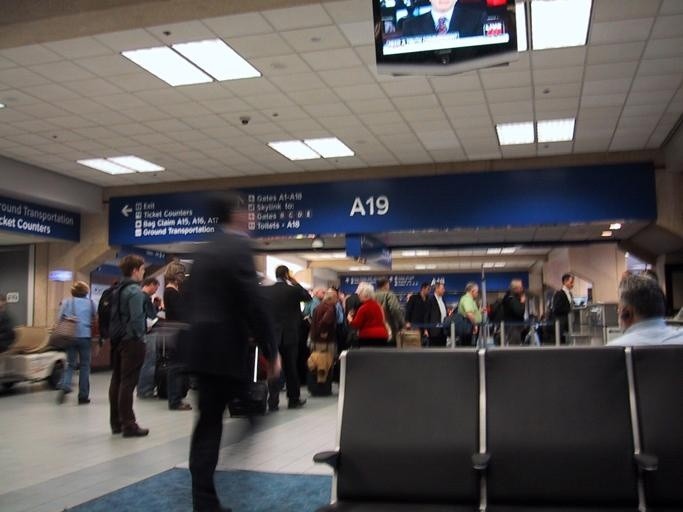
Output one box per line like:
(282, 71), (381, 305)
(371, 0), (520, 79)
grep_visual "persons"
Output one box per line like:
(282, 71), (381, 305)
(552, 273), (575, 344)
(498, 278), (526, 346)
(459, 282), (482, 346)
(401, 0), (488, 38)
(56, 280), (98, 404)
(606, 269), (683, 346)
(110, 196), (448, 512)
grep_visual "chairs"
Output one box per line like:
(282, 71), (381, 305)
(628, 345), (683, 512)
(474, 346), (656, 511)
(314, 348), (489, 511)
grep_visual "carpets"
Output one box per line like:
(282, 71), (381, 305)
(62, 467), (332, 511)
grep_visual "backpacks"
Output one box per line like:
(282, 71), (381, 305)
(491, 296), (515, 320)
(98, 280), (139, 346)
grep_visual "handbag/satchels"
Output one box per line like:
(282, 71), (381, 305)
(51, 316), (78, 350)
(443, 312), (474, 346)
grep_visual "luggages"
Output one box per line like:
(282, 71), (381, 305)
(397, 323), (422, 349)
(308, 328), (333, 396)
(228, 383), (268, 416)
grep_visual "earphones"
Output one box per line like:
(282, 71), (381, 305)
(618, 309), (631, 324)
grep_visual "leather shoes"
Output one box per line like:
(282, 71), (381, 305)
(56, 388), (307, 437)
